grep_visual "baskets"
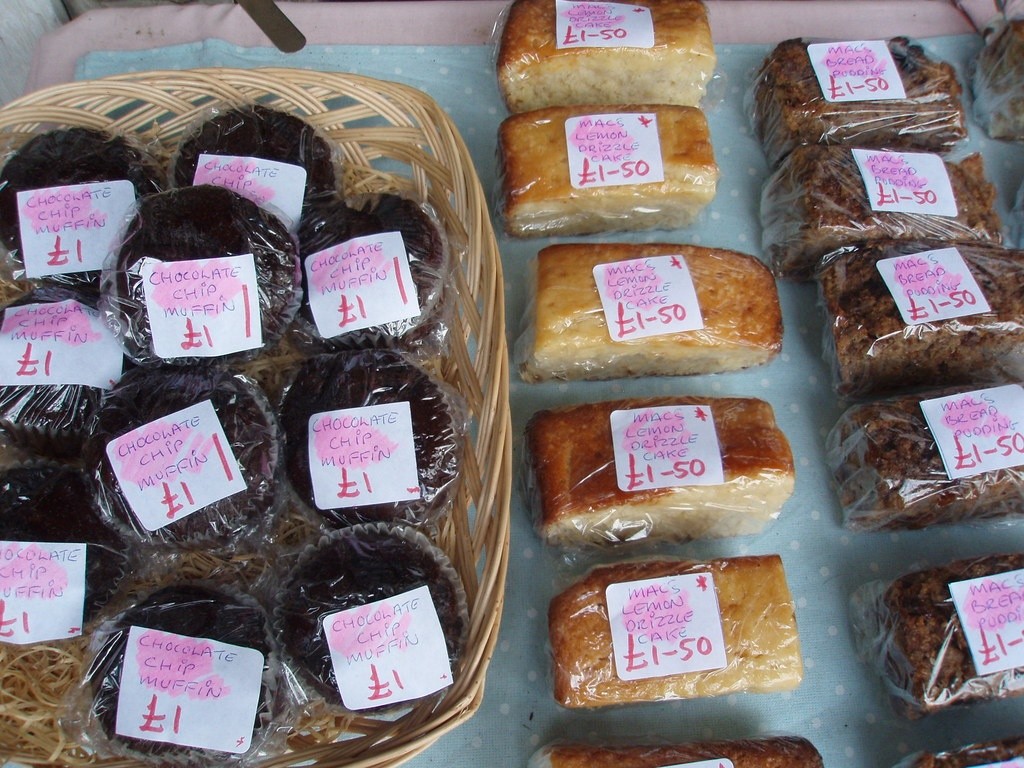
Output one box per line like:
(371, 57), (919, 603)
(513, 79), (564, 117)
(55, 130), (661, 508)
(0, 68), (513, 768)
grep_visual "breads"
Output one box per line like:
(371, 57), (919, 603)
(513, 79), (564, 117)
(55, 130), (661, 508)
(548, 554), (801, 711)
(528, 735), (824, 768)
(521, 243), (783, 375)
(496, 0), (714, 117)
(520, 395), (793, 538)
(497, 103), (717, 237)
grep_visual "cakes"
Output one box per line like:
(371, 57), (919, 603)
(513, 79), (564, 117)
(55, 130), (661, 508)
(1, 104), (465, 760)
